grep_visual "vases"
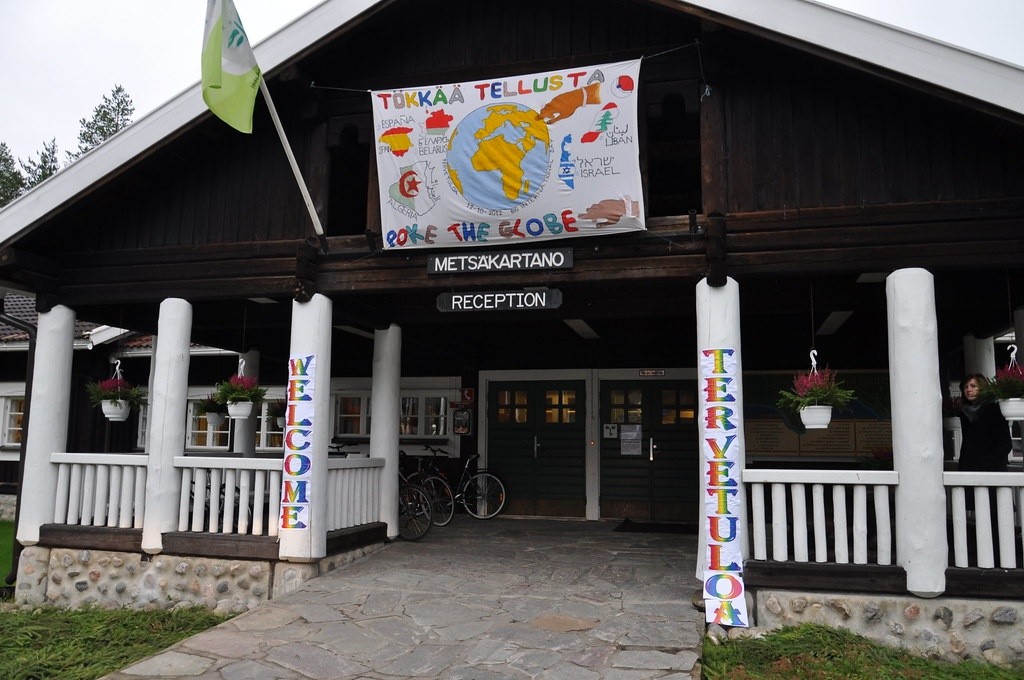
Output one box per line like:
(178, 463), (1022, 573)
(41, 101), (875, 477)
(100, 399), (131, 422)
(226, 400), (254, 420)
(996, 397), (1024, 420)
(799, 406), (832, 429)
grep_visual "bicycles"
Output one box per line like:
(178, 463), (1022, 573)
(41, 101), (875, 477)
(399, 443), (508, 520)
(327, 441), (455, 543)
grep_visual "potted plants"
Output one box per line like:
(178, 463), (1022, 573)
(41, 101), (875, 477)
(947, 363), (964, 398)
(267, 400), (288, 427)
(196, 393), (225, 426)
(942, 398), (963, 460)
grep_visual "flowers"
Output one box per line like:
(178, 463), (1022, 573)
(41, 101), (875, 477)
(86, 377), (149, 413)
(777, 368), (858, 413)
(969, 362), (1024, 403)
(211, 372), (268, 406)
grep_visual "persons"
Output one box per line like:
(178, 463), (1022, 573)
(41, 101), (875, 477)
(953, 373), (1014, 557)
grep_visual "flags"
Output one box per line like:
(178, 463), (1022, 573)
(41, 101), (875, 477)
(201, 0), (261, 135)
